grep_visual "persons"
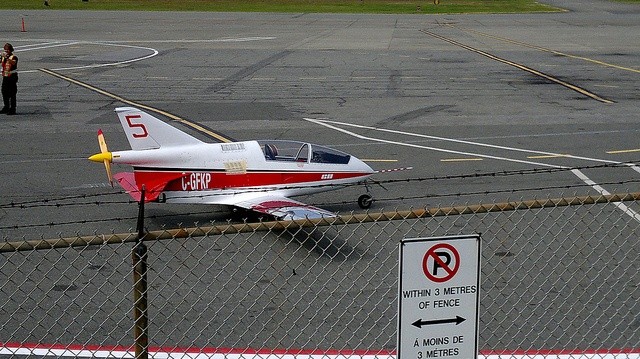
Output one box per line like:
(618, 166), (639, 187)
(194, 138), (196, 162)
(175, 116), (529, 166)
(0, 43), (18, 115)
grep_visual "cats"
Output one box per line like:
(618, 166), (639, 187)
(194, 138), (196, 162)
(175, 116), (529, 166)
(88, 106), (413, 220)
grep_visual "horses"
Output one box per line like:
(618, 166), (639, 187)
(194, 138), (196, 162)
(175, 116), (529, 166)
(1, 51), (6, 57)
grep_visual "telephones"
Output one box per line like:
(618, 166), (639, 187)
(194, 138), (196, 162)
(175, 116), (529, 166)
(396, 232), (482, 358)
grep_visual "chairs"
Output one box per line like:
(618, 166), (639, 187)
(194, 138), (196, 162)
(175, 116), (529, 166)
(6, 108), (16, 114)
(0, 106), (11, 114)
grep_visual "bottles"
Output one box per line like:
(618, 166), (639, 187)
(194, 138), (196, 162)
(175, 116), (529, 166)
(4, 43), (14, 50)
